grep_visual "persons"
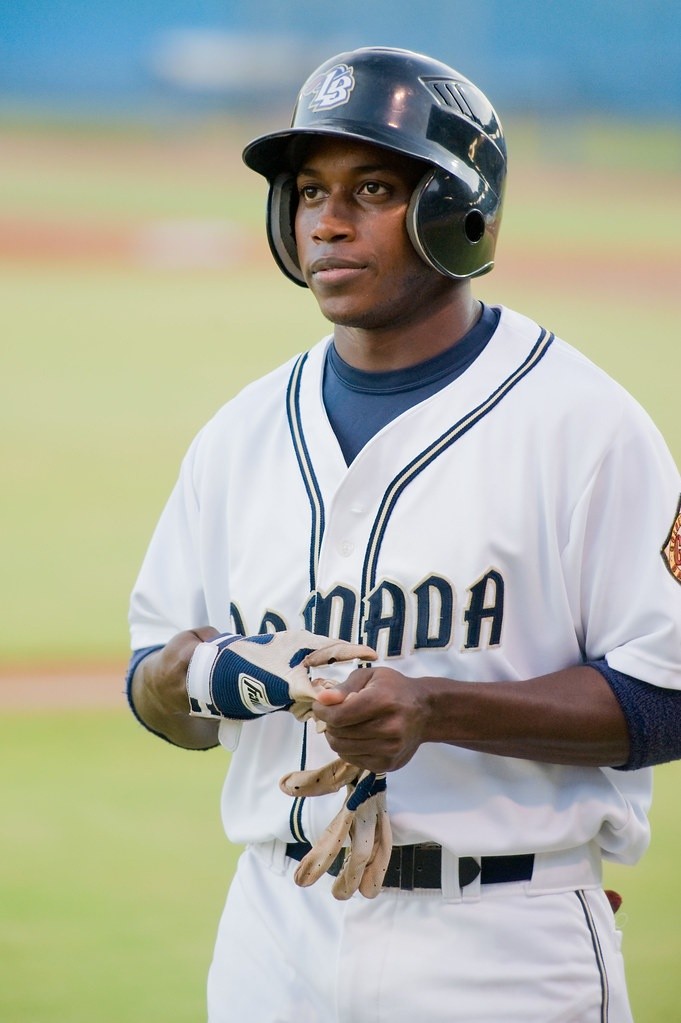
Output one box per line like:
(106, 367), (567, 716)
(120, 43), (681, 1023)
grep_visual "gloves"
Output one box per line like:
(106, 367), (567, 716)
(188, 632), (378, 734)
(280, 756), (392, 899)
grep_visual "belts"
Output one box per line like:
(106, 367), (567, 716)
(285, 834), (533, 893)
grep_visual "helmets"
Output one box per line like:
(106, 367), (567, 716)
(242, 47), (508, 282)
